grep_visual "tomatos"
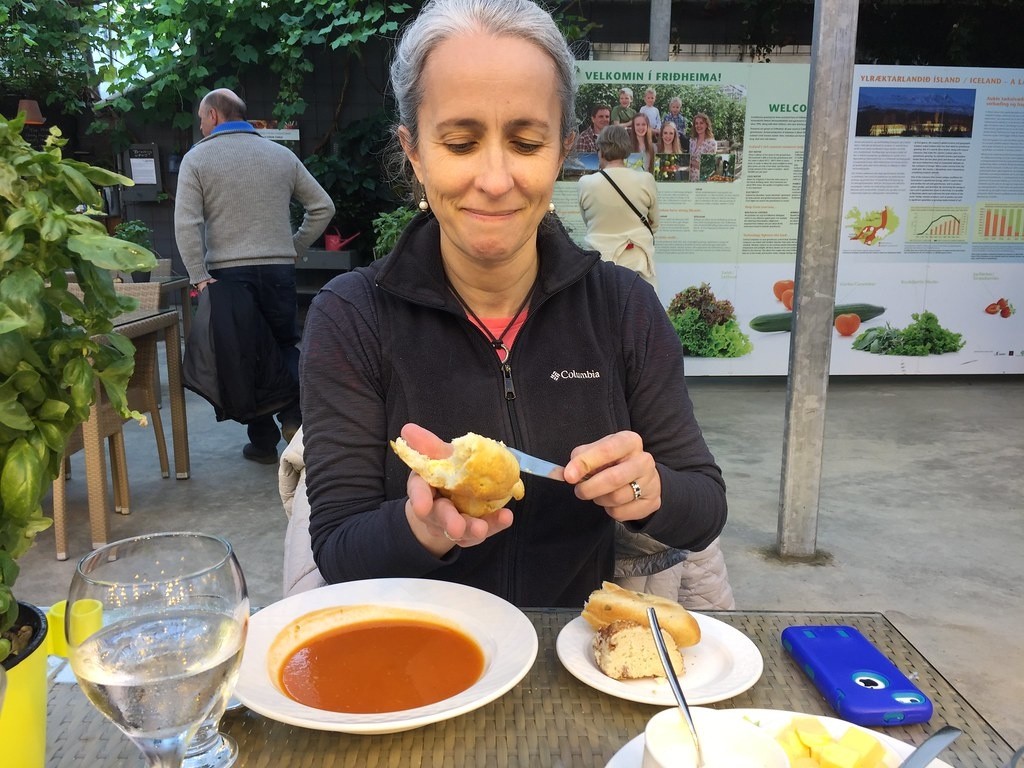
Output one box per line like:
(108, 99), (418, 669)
(835, 313), (861, 336)
(773, 280), (795, 311)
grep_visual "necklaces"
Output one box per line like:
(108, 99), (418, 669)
(446, 276), (535, 366)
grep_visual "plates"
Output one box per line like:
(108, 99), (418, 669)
(604, 708), (951, 768)
(235, 578), (538, 733)
(556, 609), (764, 706)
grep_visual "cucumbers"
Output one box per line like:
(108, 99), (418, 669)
(749, 303), (886, 332)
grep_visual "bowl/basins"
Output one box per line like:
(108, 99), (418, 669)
(642, 707), (790, 768)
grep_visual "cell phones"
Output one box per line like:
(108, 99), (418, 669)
(782, 625), (933, 726)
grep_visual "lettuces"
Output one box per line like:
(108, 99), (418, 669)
(665, 281), (753, 359)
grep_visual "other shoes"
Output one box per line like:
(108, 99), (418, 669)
(281, 418), (298, 444)
(243, 441), (278, 464)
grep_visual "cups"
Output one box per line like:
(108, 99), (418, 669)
(47, 599), (103, 658)
(151, 595), (238, 767)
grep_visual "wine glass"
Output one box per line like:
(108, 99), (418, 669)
(66, 531), (248, 768)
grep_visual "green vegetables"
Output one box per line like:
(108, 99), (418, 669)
(851, 309), (966, 357)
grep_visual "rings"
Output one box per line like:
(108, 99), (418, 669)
(629, 481), (642, 502)
(443, 529), (462, 542)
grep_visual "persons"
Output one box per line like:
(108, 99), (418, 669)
(298, 0), (726, 610)
(175, 90), (335, 467)
(576, 84), (718, 183)
(577, 126), (660, 290)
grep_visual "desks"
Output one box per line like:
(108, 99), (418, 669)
(50, 306), (192, 550)
(47, 272), (191, 410)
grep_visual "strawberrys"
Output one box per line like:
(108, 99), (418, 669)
(985, 298), (1016, 318)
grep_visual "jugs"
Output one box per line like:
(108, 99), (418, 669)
(323, 227), (361, 251)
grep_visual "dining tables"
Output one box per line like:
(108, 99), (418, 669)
(36, 607), (1024, 768)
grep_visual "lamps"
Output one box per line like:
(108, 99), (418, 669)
(17, 60), (44, 125)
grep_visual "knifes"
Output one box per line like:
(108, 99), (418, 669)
(509, 448), (588, 487)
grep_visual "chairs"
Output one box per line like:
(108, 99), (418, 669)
(41, 257), (171, 562)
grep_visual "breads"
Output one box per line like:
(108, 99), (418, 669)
(390, 432), (526, 518)
(591, 619), (685, 680)
(580, 581), (701, 649)
